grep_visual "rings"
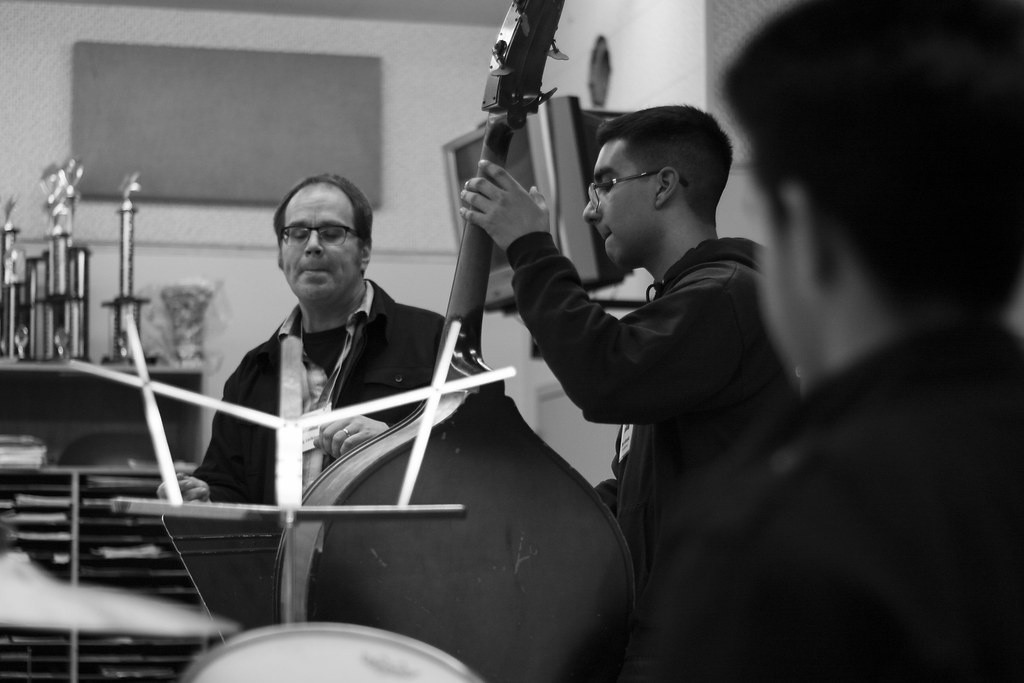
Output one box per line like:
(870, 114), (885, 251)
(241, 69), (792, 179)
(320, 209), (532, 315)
(343, 428), (351, 438)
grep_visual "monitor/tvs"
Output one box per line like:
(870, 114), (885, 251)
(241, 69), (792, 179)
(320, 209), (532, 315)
(443, 94), (634, 314)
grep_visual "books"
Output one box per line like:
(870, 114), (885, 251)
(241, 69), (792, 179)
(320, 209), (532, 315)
(0, 477), (204, 678)
(0, 433), (48, 470)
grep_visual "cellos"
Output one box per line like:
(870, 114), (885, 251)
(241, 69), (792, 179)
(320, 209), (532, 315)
(268, 0), (638, 683)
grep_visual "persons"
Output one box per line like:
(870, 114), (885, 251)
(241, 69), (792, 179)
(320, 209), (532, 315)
(157, 173), (445, 504)
(557, 0), (1024, 683)
(460, 107), (801, 594)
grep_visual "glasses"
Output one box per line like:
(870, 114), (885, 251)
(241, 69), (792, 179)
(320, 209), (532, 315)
(280, 225), (361, 247)
(588, 170), (691, 214)
